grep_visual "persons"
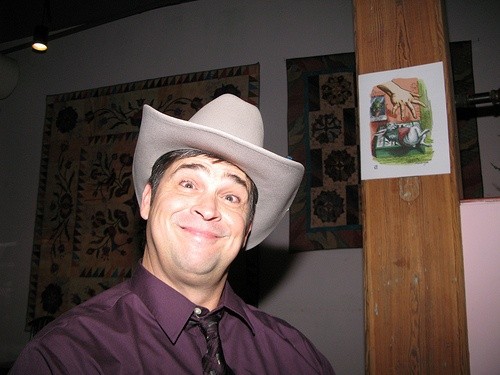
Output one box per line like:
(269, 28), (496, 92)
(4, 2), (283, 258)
(7, 94), (338, 375)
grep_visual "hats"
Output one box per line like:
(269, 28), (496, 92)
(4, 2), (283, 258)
(131, 93), (304, 250)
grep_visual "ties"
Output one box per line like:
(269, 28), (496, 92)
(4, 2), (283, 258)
(190, 307), (235, 375)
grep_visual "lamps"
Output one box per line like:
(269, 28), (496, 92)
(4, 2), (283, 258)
(31, 26), (48, 52)
